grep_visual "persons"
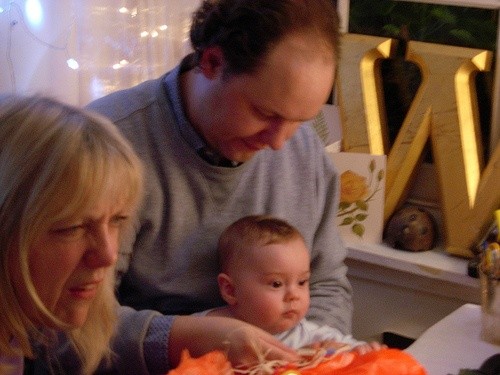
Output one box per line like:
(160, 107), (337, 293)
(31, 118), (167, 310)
(0, 94), (142, 375)
(77, 1), (355, 372)
(178, 210), (390, 367)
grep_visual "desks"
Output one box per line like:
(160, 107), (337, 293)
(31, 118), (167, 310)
(344, 199), (499, 352)
(403, 302), (500, 375)
